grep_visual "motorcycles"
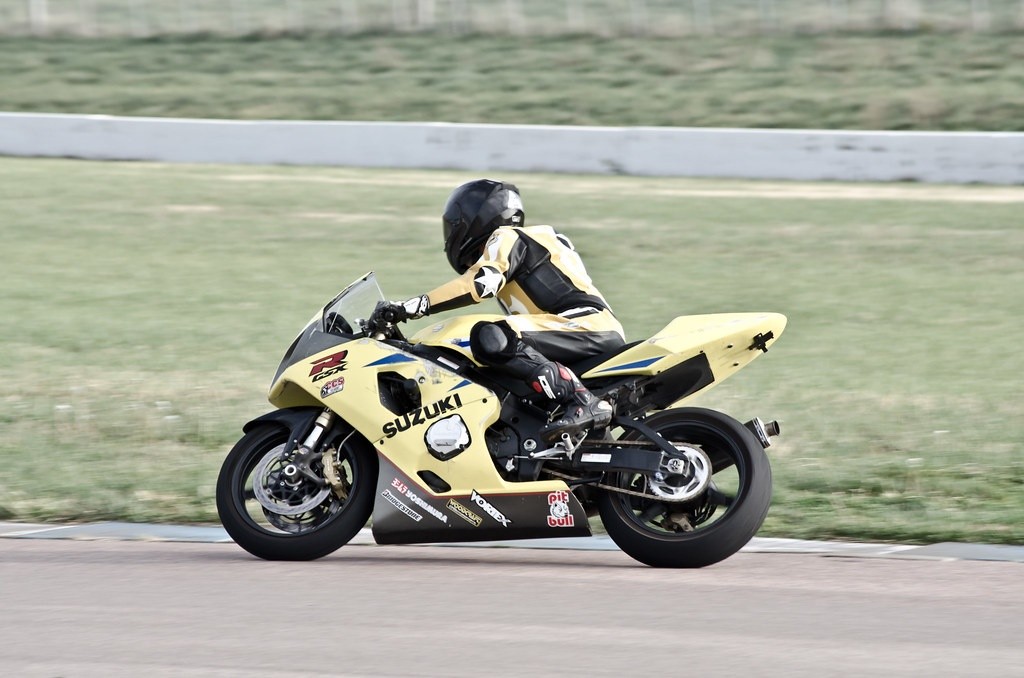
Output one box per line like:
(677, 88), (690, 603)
(213, 267), (791, 571)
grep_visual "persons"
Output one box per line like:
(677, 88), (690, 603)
(366, 178), (626, 444)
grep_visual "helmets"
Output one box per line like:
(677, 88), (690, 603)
(443, 178), (525, 274)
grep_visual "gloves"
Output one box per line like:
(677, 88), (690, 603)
(369, 294), (431, 333)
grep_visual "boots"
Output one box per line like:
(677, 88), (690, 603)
(527, 360), (612, 443)
